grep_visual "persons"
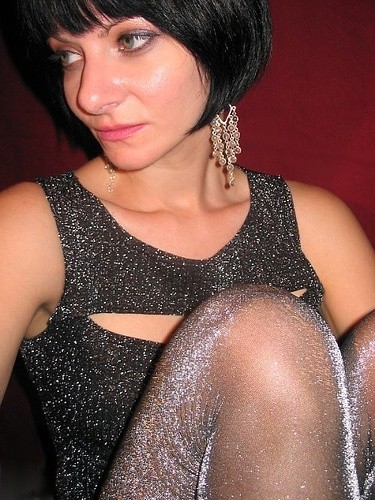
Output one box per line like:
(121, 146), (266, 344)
(0, 0), (375, 500)
